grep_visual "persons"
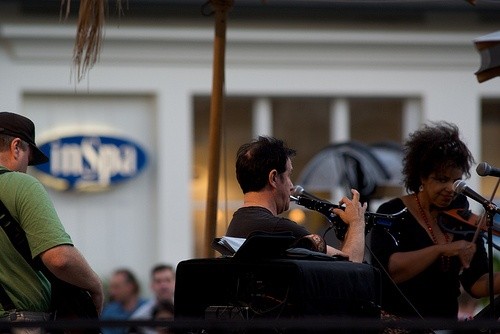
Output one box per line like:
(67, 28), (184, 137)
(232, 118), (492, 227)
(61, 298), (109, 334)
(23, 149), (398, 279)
(144, 301), (175, 334)
(131, 262), (178, 321)
(101, 269), (153, 334)
(1, 111), (105, 334)
(370, 120), (500, 334)
(226, 133), (370, 264)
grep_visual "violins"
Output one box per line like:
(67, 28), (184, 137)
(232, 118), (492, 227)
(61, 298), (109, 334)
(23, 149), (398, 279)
(446, 207), (500, 237)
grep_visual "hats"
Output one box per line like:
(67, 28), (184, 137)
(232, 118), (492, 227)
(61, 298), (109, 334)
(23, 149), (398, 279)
(0, 112), (50, 166)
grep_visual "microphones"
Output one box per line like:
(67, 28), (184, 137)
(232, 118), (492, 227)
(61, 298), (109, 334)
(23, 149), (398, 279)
(476, 162), (500, 178)
(453, 180), (500, 215)
(292, 185), (323, 202)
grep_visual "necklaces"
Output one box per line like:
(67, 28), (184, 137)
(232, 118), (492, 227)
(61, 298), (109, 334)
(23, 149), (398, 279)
(414, 193), (451, 261)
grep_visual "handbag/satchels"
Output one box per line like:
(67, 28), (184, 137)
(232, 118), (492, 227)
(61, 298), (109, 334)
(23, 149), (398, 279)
(50, 273), (101, 334)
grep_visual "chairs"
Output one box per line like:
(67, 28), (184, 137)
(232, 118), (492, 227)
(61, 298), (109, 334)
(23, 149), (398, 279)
(174, 258), (382, 334)
